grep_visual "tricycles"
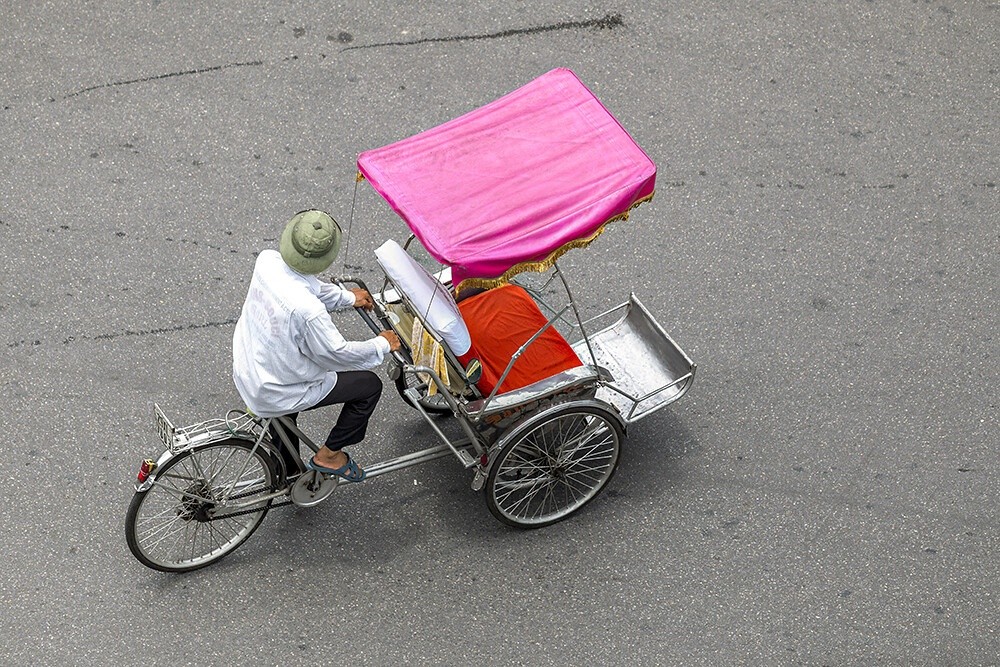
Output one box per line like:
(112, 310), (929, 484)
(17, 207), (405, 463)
(121, 69), (694, 574)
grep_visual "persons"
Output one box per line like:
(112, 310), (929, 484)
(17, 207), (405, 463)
(232, 209), (402, 483)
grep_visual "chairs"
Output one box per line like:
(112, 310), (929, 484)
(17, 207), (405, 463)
(375, 238), (615, 417)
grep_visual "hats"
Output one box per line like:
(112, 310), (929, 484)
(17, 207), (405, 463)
(281, 209), (343, 275)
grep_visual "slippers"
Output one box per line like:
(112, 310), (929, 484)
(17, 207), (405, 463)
(309, 452), (366, 483)
